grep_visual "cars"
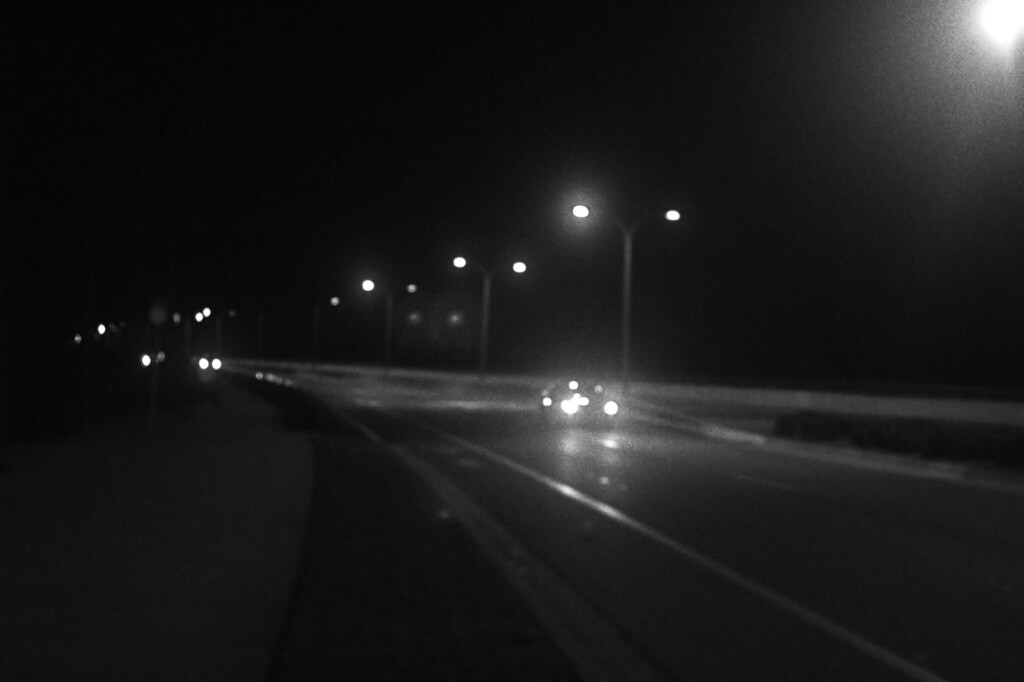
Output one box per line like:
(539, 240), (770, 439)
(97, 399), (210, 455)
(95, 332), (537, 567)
(541, 379), (617, 426)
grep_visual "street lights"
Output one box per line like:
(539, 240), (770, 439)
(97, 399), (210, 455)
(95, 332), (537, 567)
(453, 257), (527, 374)
(363, 280), (418, 370)
(573, 203), (681, 383)
